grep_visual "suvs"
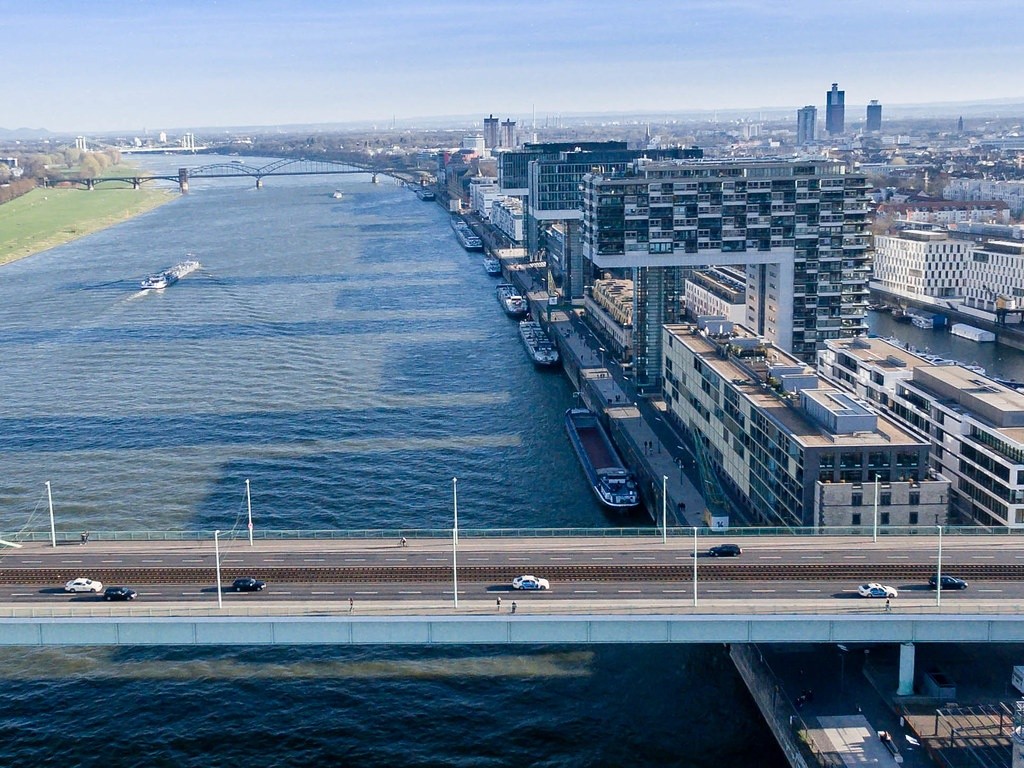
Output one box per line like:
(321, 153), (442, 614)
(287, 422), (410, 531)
(709, 544), (742, 557)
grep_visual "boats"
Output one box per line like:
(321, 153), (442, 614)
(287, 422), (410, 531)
(406, 181), (438, 201)
(497, 282), (530, 316)
(565, 406), (641, 509)
(519, 319), (560, 366)
(482, 256), (503, 275)
(141, 261), (197, 288)
(450, 216), (483, 250)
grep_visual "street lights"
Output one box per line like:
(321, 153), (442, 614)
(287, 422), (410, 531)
(44, 481), (57, 548)
(611, 361), (615, 389)
(873, 473), (882, 542)
(600, 348), (605, 367)
(244, 477), (256, 547)
(589, 334), (593, 360)
(579, 321), (583, 345)
(452, 527), (458, 608)
(663, 475), (669, 544)
(453, 477), (462, 545)
(656, 418), (662, 453)
(691, 527), (699, 609)
(678, 445), (685, 484)
(215, 530), (223, 609)
(624, 376), (629, 403)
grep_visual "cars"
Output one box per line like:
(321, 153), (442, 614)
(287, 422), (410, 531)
(232, 576), (266, 593)
(103, 586), (137, 601)
(930, 575), (969, 590)
(858, 584), (898, 598)
(512, 576), (550, 591)
(65, 577), (103, 594)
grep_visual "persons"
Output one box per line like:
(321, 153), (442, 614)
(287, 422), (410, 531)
(511, 601), (516, 612)
(497, 596), (501, 605)
(79, 530), (90, 545)
(348, 597), (353, 612)
(400, 537), (407, 547)
(884, 598), (891, 611)
(482, 228), (686, 511)
